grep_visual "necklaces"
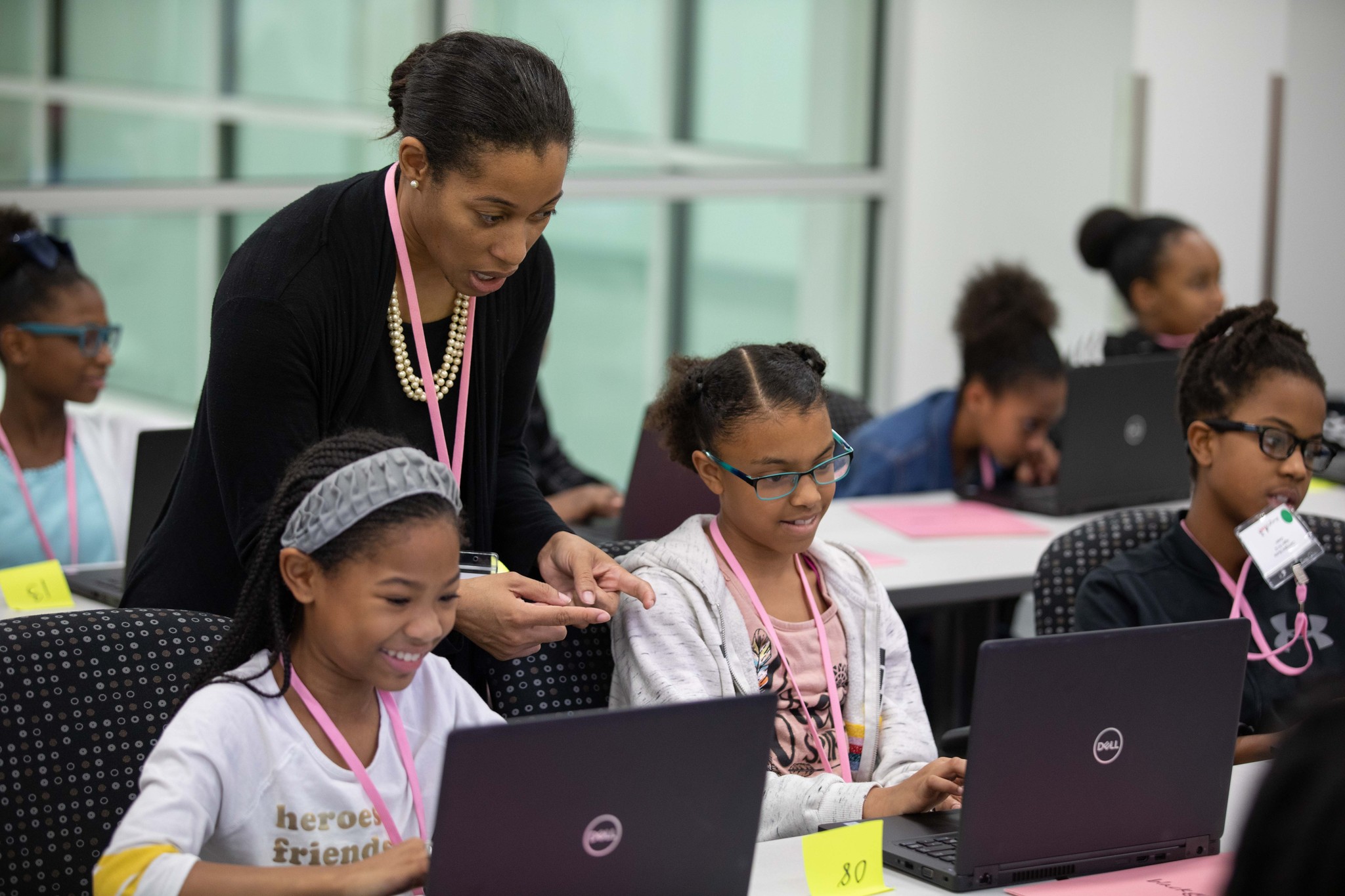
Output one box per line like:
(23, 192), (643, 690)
(387, 282), (470, 401)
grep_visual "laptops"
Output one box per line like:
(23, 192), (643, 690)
(565, 403), (721, 547)
(65, 429), (195, 610)
(818, 618), (1253, 891)
(950, 356), (1189, 518)
(425, 693), (778, 896)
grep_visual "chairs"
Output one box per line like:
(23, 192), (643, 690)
(0, 607), (257, 896)
(479, 537), (671, 715)
(1033, 504), (1345, 636)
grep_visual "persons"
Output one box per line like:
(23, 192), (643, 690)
(1074, 300), (1345, 765)
(833, 264), (1068, 499)
(1079, 208), (1223, 361)
(119, 32), (656, 660)
(92, 430), (508, 896)
(0, 206), (122, 569)
(610, 342), (967, 843)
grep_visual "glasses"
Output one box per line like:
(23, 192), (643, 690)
(1204, 419), (1337, 473)
(702, 429), (854, 501)
(16, 325), (122, 357)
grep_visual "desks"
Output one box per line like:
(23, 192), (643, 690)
(747, 760), (1272, 896)
(0, 477), (1344, 672)
